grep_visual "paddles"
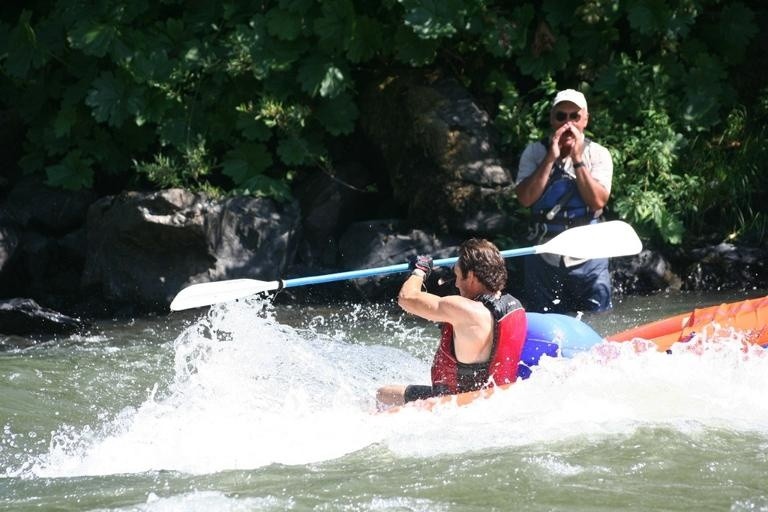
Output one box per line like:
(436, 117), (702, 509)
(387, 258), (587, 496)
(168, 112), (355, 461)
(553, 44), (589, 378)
(169, 220), (644, 312)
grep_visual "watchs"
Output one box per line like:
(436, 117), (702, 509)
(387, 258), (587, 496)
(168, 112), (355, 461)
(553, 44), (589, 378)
(409, 268), (426, 280)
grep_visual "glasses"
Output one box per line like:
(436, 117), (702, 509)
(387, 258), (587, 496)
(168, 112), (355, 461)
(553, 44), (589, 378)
(551, 109), (582, 122)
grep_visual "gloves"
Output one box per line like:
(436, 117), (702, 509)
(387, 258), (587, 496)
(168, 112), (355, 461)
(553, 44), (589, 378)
(408, 253), (434, 282)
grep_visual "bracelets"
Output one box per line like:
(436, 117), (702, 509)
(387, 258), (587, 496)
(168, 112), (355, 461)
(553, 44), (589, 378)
(572, 160), (584, 168)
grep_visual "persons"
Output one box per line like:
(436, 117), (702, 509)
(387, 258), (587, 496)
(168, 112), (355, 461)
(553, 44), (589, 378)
(516, 88), (615, 312)
(376, 238), (529, 407)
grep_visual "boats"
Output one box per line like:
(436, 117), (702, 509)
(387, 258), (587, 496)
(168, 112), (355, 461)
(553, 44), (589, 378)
(377, 294), (768, 422)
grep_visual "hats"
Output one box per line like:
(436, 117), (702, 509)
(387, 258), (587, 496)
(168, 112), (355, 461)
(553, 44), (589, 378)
(551, 88), (588, 114)
